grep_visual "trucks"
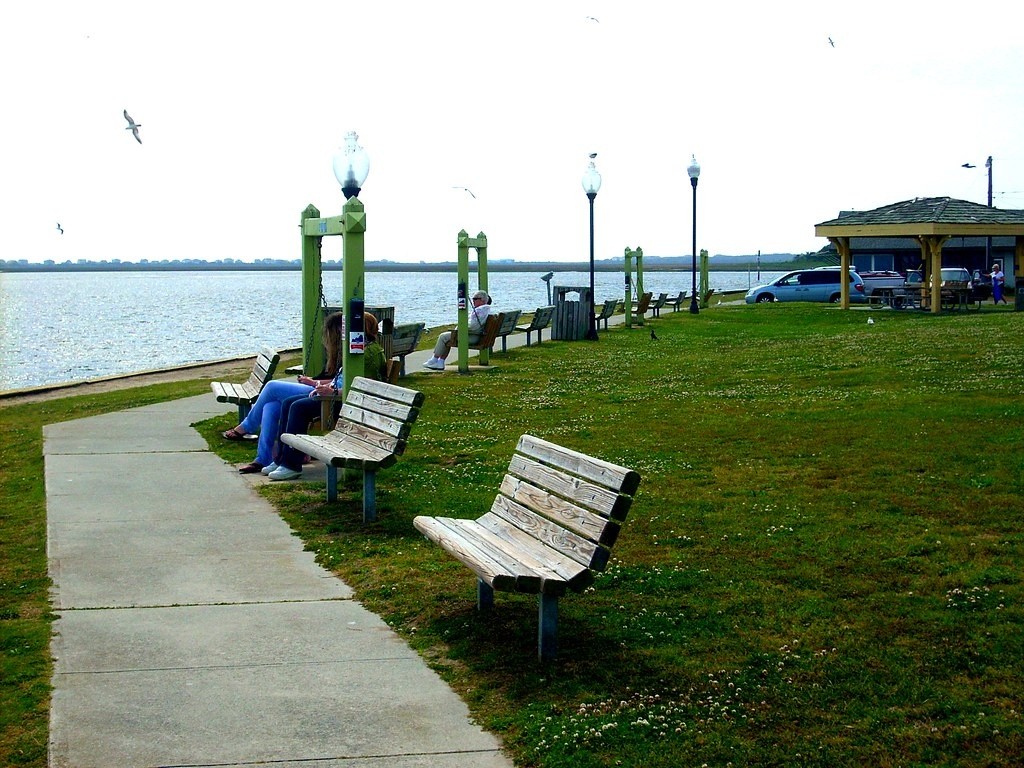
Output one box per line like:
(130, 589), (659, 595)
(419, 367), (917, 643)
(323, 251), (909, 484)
(814, 265), (904, 306)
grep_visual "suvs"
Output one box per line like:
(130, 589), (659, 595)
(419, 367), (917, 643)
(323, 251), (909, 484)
(744, 268), (865, 305)
(903, 266), (989, 307)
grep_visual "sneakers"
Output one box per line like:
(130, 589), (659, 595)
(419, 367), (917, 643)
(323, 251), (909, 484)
(261, 461), (303, 480)
(423, 361), (445, 370)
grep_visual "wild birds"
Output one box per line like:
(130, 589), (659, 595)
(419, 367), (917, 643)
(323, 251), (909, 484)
(56, 223), (64, 235)
(453, 186), (476, 200)
(123, 109), (143, 145)
(828, 38), (836, 48)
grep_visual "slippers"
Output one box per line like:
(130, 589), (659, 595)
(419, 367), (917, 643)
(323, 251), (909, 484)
(238, 461), (265, 474)
(221, 427), (258, 440)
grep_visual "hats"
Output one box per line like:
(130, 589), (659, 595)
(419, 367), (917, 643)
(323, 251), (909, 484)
(992, 264), (999, 269)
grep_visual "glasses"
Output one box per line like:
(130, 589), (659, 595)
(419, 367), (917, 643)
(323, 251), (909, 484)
(472, 297), (482, 300)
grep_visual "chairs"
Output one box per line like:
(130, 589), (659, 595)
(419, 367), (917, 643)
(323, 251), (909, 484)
(972, 284), (992, 310)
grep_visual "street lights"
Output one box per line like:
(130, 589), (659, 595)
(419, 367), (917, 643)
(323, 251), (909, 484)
(582, 161), (601, 339)
(688, 153), (702, 316)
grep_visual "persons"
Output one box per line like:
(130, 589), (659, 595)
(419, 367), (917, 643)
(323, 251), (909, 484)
(221, 311), (389, 481)
(422, 290), (495, 372)
(797, 274), (801, 284)
(488, 294), (493, 306)
(981, 264), (1009, 305)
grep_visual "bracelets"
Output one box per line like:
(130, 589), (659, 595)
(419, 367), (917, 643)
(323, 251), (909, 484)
(334, 388), (339, 395)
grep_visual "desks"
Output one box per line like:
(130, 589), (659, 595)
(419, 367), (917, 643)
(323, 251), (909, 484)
(872, 285), (925, 310)
(938, 287), (973, 312)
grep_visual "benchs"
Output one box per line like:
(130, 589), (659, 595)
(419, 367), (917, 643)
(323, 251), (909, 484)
(490, 309), (522, 355)
(647, 293), (667, 318)
(448, 311), (505, 352)
(940, 294), (954, 310)
(210, 344), (280, 441)
(863, 294), (894, 308)
(617, 292), (653, 315)
(595, 298), (619, 331)
(695, 288), (715, 301)
(410, 432), (641, 662)
(285, 354), (401, 436)
(516, 305), (556, 347)
(895, 291), (922, 309)
(279, 377), (424, 522)
(391, 320), (426, 374)
(665, 291), (687, 312)
